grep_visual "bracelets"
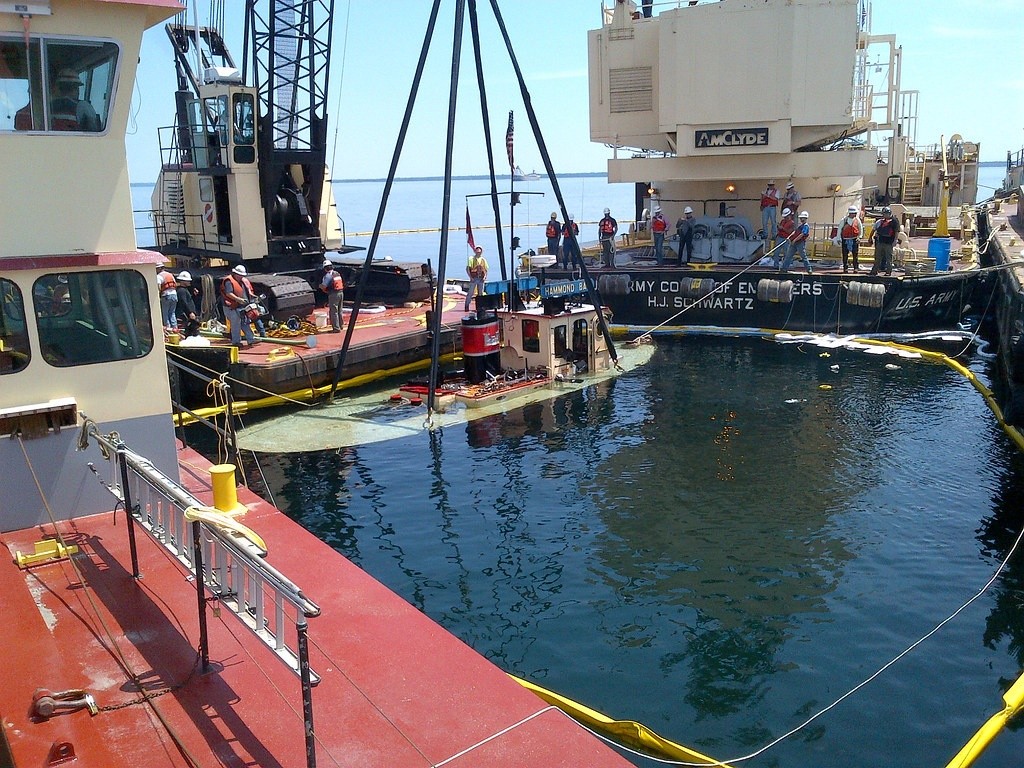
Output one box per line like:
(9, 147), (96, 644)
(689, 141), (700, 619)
(793, 201), (795, 205)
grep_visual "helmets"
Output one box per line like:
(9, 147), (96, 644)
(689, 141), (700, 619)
(767, 179), (809, 220)
(882, 207), (892, 214)
(684, 207), (692, 213)
(56, 68), (84, 87)
(474, 245), (483, 251)
(156, 262), (164, 268)
(232, 264), (246, 275)
(551, 212), (557, 217)
(848, 206), (858, 213)
(569, 213), (574, 220)
(57, 275), (68, 283)
(604, 208), (610, 214)
(176, 271), (192, 280)
(653, 205), (661, 213)
(322, 259), (332, 268)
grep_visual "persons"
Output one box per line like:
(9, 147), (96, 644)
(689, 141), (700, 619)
(770, 208), (813, 274)
(320, 261), (344, 334)
(649, 206), (671, 266)
(674, 206), (696, 268)
(464, 245), (489, 312)
(175, 271), (199, 325)
(561, 213), (579, 271)
(546, 212), (561, 269)
(642, 0), (653, 18)
(156, 262), (178, 331)
(50, 67), (103, 132)
(14, 86), (32, 130)
(221, 265), (266, 347)
(836, 205), (863, 274)
(781, 181), (801, 227)
(54, 275), (69, 310)
(866, 207), (900, 276)
(598, 208), (618, 269)
(760, 179), (780, 240)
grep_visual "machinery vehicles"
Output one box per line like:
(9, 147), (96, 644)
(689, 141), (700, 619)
(137, 0), (438, 322)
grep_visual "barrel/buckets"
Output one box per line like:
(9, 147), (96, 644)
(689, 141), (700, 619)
(927, 238), (952, 271)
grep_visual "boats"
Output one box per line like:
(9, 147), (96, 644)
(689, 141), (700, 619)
(227, 109), (654, 452)
(465, 376), (617, 454)
(515, 0), (980, 336)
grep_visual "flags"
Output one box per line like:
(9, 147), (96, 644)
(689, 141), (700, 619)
(506, 110), (515, 169)
(466, 205), (475, 250)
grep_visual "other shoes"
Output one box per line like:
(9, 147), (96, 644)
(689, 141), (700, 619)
(613, 264), (618, 269)
(249, 340), (262, 344)
(844, 268), (848, 273)
(884, 272), (891, 276)
(808, 267), (813, 274)
(854, 269), (859, 273)
(235, 342), (244, 347)
(866, 271), (877, 275)
(327, 328), (340, 333)
(465, 304), (469, 312)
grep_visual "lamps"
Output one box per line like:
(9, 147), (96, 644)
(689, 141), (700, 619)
(831, 183), (841, 191)
(727, 185), (735, 193)
(648, 188), (658, 195)
